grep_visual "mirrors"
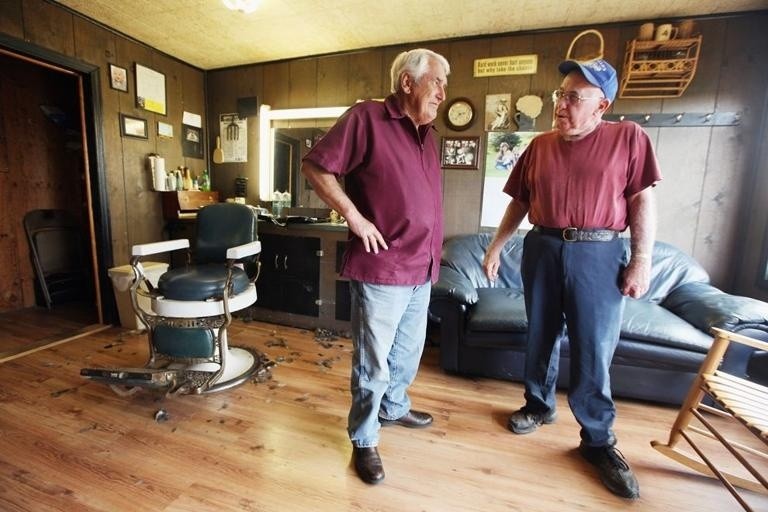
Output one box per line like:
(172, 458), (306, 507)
(259, 104), (351, 219)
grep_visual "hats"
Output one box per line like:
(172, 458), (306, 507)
(558, 56), (619, 101)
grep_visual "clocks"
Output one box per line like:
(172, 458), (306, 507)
(442, 96), (476, 132)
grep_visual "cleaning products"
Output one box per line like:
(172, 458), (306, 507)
(282, 189), (292, 208)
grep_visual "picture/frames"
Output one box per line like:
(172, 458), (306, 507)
(438, 134), (481, 170)
(119, 112), (149, 141)
(134, 60), (168, 116)
(108, 62), (129, 92)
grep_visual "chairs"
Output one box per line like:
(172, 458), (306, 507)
(650, 327), (768, 512)
(80, 202), (262, 398)
(23, 208), (79, 310)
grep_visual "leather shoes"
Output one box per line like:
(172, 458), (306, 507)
(352, 441), (386, 484)
(377, 407), (434, 429)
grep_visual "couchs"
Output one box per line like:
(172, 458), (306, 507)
(427, 233), (768, 411)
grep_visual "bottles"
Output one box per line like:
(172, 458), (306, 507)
(282, 189), (291, 208)
(168, 166), (209, 191)
(272, 189), (283, 217)
(330, 209), (339, 222)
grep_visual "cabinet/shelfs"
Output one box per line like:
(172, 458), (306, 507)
(161, 191), (352, 337)
(617, 35), (702, 99)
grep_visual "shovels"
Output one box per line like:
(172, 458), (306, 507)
(213, 137), (224, 164)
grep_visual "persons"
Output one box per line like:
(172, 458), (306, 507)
(498, 141), (513, 167)
(297, 47), (452, 488)
(484, 57), (662, 502)
(489, 96), (508, 131)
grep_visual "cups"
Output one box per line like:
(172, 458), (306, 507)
(639, 18), (697, 40)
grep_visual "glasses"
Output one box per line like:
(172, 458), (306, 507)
(551, 90), (604, 104)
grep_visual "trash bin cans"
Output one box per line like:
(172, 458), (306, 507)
(107, 261), (169, 331)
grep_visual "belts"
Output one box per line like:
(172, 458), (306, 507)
(532, 223), (619, 244)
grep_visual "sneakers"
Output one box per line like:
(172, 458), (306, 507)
(506, 406), (558, 433)
(575, 438), (640, 498)
